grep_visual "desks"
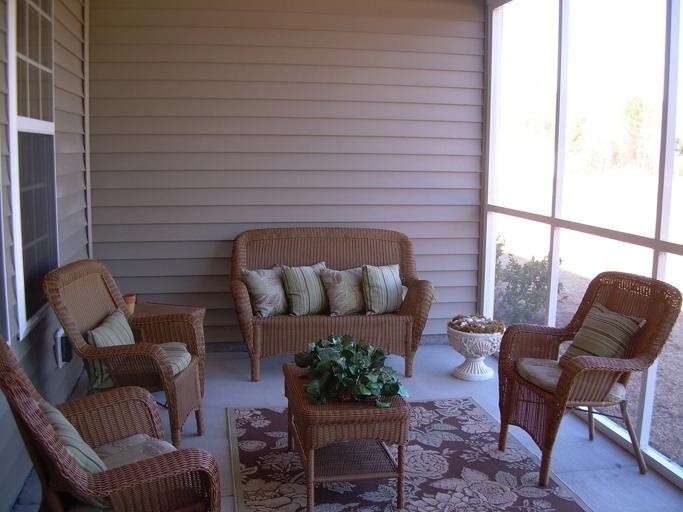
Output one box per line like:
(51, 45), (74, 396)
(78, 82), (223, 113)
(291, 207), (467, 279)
(130, 301), (207, 415)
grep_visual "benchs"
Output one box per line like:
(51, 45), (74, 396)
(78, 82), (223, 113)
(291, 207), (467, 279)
(231, 227), (433, 382)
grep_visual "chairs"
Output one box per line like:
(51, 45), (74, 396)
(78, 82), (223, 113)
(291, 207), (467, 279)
(44, 259), (207, 448)
(1, 337), (222, 512)
(499, 272), (682, 486)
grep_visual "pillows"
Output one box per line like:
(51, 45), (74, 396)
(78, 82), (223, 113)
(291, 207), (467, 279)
(559, 302), (648, 370)
(87, 310), (134, 387)
(241, 261), (409, 317)
(42, 401), (106, 476)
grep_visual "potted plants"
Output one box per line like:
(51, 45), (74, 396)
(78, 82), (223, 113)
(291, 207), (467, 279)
(295, 333), (401, 408)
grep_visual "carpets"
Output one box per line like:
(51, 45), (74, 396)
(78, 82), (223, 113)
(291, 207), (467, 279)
(226, 396), (595, 512)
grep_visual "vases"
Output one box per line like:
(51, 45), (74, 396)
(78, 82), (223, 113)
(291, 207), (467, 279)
(447, 321), (506, 382)
